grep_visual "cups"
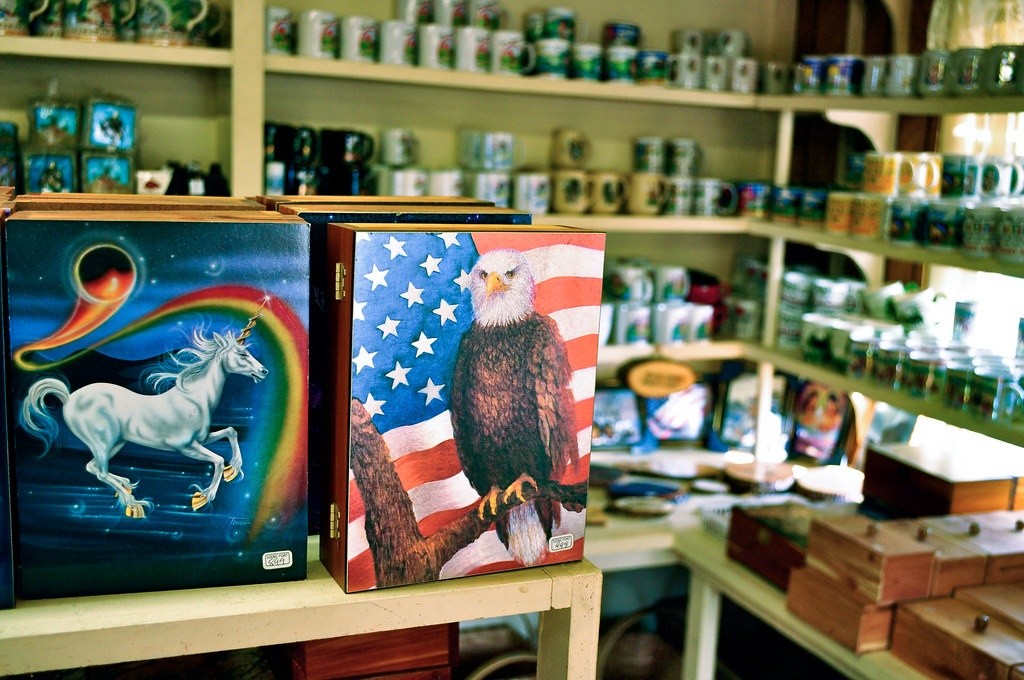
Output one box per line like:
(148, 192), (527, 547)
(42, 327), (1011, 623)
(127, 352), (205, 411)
(0, 1), (1023, 425)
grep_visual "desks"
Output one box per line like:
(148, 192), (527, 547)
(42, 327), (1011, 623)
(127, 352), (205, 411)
(676, 527), (933, 680)
(0, 557), (603, 680)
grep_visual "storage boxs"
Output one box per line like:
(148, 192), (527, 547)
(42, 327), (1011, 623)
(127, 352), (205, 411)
(724, 441), (1024, 680)
(289, 622), (461, 680)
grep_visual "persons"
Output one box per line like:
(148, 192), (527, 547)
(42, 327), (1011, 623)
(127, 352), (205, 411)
(804, 391), (840, 432)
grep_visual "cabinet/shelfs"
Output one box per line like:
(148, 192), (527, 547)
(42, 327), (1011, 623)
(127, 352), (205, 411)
(0, 1), (1024, 572)
